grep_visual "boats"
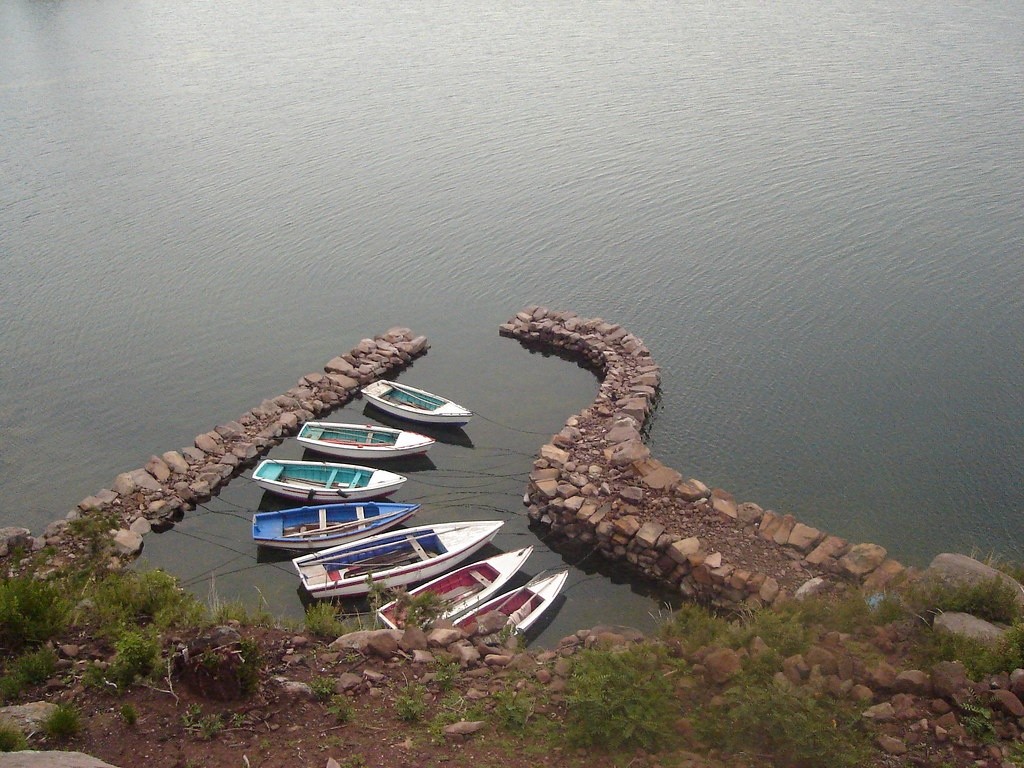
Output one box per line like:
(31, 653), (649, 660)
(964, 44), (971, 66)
(252, 459), (409, 507)
(297, 420), (437, 462)
(451, 570), (570, 643)
(290, 519), (506, 600)
(374, 545), (537, 634)
(250, 501), (423, 555)
(360, 378), (476, 428)
